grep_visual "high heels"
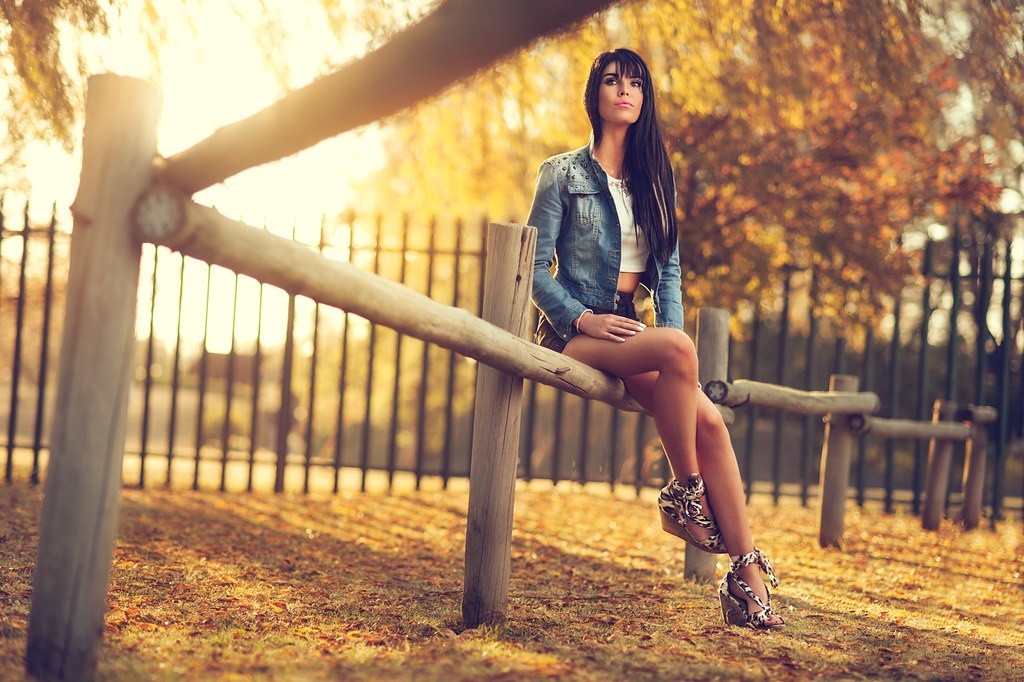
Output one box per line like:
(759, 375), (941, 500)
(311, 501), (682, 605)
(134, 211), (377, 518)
(717, 546), (784, 631)
(658, 473), (728, 554)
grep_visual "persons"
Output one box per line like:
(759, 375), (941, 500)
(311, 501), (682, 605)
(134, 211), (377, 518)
(527, 48), (784, 630)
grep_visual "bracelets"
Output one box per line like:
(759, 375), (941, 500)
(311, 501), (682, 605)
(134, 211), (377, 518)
(577, 309), (593, 333)
